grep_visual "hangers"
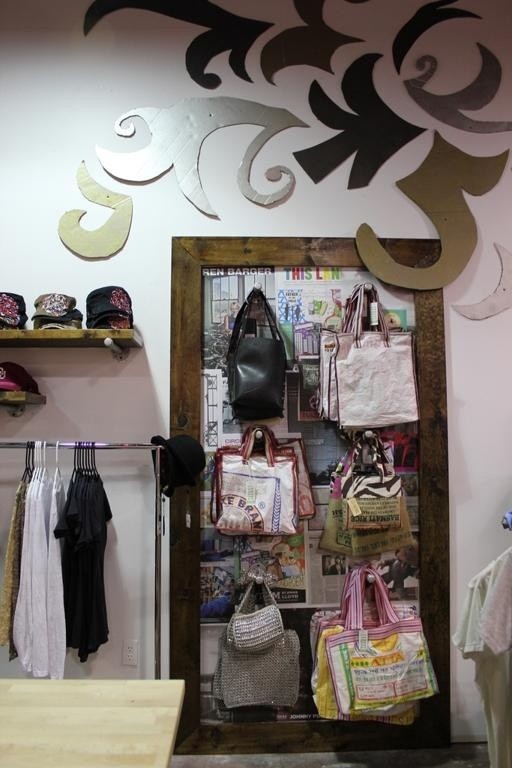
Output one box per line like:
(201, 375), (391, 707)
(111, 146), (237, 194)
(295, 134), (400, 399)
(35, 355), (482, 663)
(18, 439), (104, 488)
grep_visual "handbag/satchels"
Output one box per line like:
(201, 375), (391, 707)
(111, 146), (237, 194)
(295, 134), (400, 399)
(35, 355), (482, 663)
(228, 289), (286, 420)
(308, 566), (441, 727)
(209, 424), (315, 537)
(317, 435), (414, 556)
(314, 284), (419, 431)
(211, 564), (301, 726)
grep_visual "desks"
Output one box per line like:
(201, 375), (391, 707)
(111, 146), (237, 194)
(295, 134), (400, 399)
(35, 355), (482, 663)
(2, 676), (191, 767)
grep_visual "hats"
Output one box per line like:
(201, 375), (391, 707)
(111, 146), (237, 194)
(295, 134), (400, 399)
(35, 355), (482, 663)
(0, 286), (28, 329)
(86, 286), (135, 329)
(0, 361), (39, 394)
(31, 293), (83, 332)
(150, 434), (205, 496)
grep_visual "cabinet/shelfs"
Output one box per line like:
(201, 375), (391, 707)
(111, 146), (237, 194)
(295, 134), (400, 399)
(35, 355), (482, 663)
(0, 325), (147, 414)
